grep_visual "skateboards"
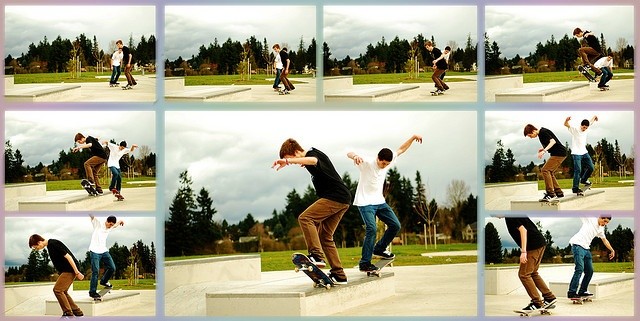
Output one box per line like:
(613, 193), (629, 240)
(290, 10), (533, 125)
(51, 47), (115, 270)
(273, 85), (290, 95)
(109, 83), (120, 87)
(121, 85), (133, 91)
(429, 90), (445, 96)
(599, 82), (610, 91)
(571, 295), (594, 304)
(539, 195), (565, 206)
(290, 252), (335, 290)
(576, 63), (597, 82)
(577, 182), (593, 196)
(112, 189), (124, 201)
(81, 179), (99, 196)
(93, 283), (113, 302)
(512, 303), (556, 316)
(367, 254), (395, 277)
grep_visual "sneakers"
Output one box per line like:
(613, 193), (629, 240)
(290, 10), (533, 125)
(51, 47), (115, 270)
(542, 192), (558, 200)
(444, 86), (450, 90)
(434, 88), (445, 93)
(100, 281), (114, 288)
(281, 88), (291, 91)
(307, 253), (327, 268)
(554, 190), (565, 197)
(359, 263), (380, 271)
(573, 188), (584, 192)
(372, 248), (395, 259)
(542, 297), (558, 308)
(580, 179), (593, 185)
(126, 83), (132, 86)
(326, 271), (348, 284)
(522, 299), (545, 310)
(132, 81), (137, 85)
(567, 291), (582, 299)
(59, 313), (76, 320)
(577, 291), (594, 297)
(582, 63), (592, 70)
(89, 291), (102, 299)
(291, 87), (296, 90)
(95, 190), (104, 194)
(594, 71), (603, 78)
(88, 181), (96, 185)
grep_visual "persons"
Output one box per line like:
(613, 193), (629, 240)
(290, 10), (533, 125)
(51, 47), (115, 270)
(110, 47), (123, 84)
(29, 233), (84, 316)
(89, 215), (124, 298)
(489, 211), (557, 309)
(573, 27), (603, 77)
(564, 115), (598, 192)
(73, 132), (106, 195)
(273, 43), (295, 91)
(424, 40), (449, 92)
(273, 47), (287, 88)
(272, 138), (351, 284)
(102, 139), (139, 193)
(524, 123), (568, 201)
(568, 214), (614, 297)
(347, 134), (422, 270)
(116, 39), (137, 87)
(432, 45), (451, 86)
(593, 52), (613, 87)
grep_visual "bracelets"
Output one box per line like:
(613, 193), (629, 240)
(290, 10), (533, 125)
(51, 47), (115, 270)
(286, 158), (289, 165)
